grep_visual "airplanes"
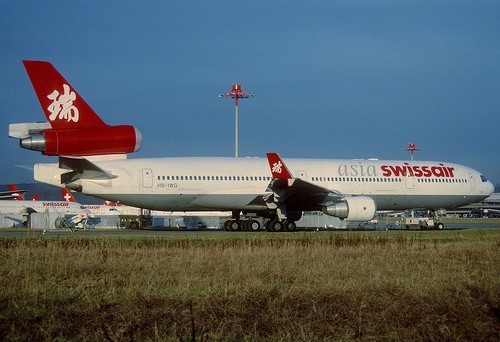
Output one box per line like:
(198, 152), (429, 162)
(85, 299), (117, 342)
(8, 60), (496, 232)
